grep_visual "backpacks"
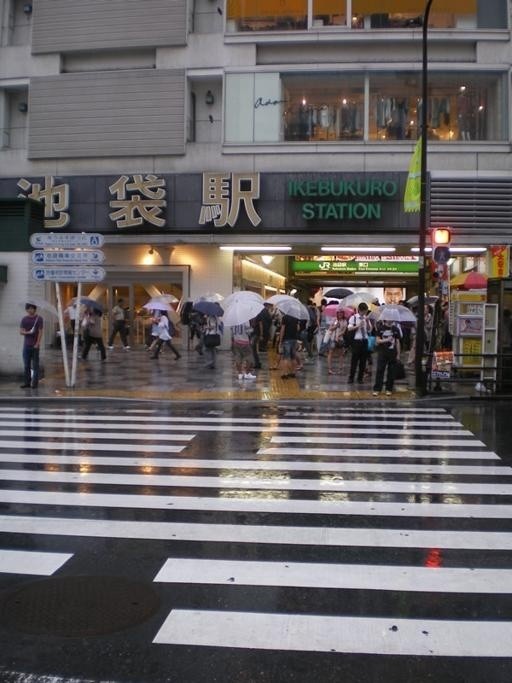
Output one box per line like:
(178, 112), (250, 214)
(165, 317), (176, 337)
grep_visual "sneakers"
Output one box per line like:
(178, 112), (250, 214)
(106, 345), (114, 349)
(372, 390), (380, 396)
(20, 384), (38, 389)
(237, 373), (257, 380)
(123, 345), (131, 350)
(174, 355), (181, 360)
(385, 389), (393, 396)
(77, 355), (86, 362)
(150, 356), (159, 360)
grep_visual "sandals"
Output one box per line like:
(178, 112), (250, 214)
(328, 368), (344, 375)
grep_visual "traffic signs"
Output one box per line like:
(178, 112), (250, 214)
(32, 250), (105, 266)
(33, 266), (105, 284)
(30, 232), (103, 248)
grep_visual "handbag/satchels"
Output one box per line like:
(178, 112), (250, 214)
(234, 339), (249, 348)
(367, 332), (376, 352)
(390, 358), (407, 380)
(342, 315), (356, 349)
(203, 334), (221, 348)
(323, 329), (333, 343)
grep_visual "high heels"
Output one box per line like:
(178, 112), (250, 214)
(281, 373), (296, 379)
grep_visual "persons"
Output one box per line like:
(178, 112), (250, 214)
(21, 303), (43, 387)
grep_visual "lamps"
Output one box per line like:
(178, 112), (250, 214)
(18, 101), (27, 112)
(205, 89), (214, 105)
(23, 4), (32, 14)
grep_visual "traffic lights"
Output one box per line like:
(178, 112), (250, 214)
(430, 224), (451, 265)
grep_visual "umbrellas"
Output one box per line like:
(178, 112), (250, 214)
(17, 297), (58, 327)
(322, 288), (354, 298)
(338, 292), (377, 308)
(447, 270), (492, 291)
(193, 291), (223, 302)
(151, 292), (181, 303)
(406, 293), (439, 304)
(224, 289), (265, 305)
(265, 294), (294, 305)
